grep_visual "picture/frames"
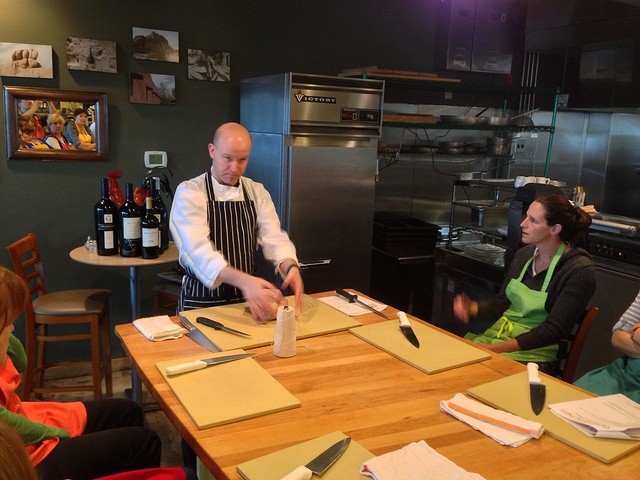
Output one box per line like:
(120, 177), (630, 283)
(3, 86), (110, 160)
(0, 42), (54, 79)
(66, 37), (119, 74)
(129, 71), (176, 105)
(188, 48), (232, 82)
(132, 27), (180, 62)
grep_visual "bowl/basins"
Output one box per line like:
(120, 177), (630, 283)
(438, 114), (490, 130)
(508, 115), (536, 127)
(408, 145), (441, 155)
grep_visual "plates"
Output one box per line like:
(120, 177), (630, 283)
(466, 244), (506, 268)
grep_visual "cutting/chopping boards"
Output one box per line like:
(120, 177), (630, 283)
(154, 349), (302, 430)
(237, 430), (377, 480)
(341, 68), (439, 78)
(348, 317), (492, 375)
(179, 291), (362, 352)
(466, 363), (640, 464)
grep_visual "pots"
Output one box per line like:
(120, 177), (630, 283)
(486, 135), (522, 156)
(479, 107), (540, 127)
(463, 142), (497, 154)
(439, 139), (486, 155)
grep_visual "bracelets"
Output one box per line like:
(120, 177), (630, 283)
(630, 327), (639, 339)
(285, 263), (301, 273)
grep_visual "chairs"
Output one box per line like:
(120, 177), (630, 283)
(558, 304), (600, 380)
(6, 233), (113, 403)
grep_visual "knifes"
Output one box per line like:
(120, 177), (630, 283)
(396, 311), (421, 348)
(277, 436), (353, 480)
(197, 315), (254, 337)
(334, 287), (390, 319)
(527, 360), (546, 418)
(179, 316), (220, 354)
(164, 352), (258, 376)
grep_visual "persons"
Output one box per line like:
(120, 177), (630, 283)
(17, 99), (96, 149)
(570, 290), (640, 403)
(0, 333), (162, 479)
(0, 264), (201, 480)
(168, 122), (304, 326)
(453, 194), (598, 374)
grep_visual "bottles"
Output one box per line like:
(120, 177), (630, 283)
(93, 178), (122, 255)
(140, 178), (165, 255)
(140, 196), (161, 259)
(151, 176), (169, 251)
(119, 183), (142, 258)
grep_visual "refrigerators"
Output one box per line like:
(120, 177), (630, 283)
(238, 73), (386, 296)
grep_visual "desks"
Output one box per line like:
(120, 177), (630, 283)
(69, 238), (178, 410)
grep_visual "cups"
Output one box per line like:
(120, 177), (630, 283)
(535, 176), (551, 184)
